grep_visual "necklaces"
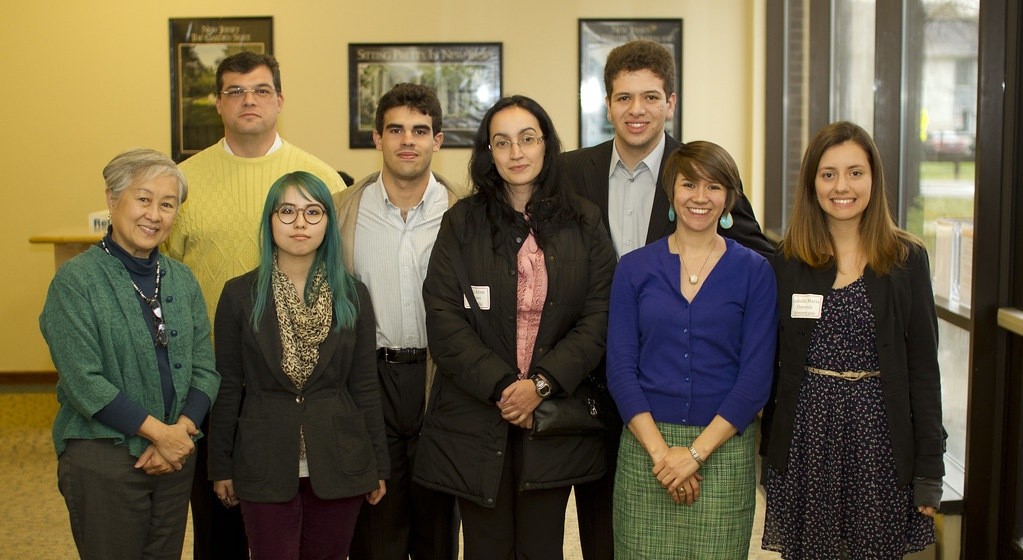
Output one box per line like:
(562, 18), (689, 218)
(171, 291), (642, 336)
(675, 234), (717, 284)
(101, 239), (168, 350)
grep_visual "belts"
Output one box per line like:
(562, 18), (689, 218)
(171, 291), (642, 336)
(377, 347), (427, 365)
(803, 365), (880, 381)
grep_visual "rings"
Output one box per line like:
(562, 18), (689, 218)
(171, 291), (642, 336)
(678, 488), (684, 493)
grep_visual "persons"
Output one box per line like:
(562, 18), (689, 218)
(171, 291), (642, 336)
(158, 51), (348, 560)
(606, 142), (778, 560)
(561, 40), (775, 560)
(413, 95), (618, 560)
(759, 120), (949, 560)
(208, 170), (390, 560)
(331, 83), (472, 560)
(39, 149), (221, 560)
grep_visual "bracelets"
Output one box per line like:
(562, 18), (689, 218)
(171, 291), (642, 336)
(688, 444), (704, 466)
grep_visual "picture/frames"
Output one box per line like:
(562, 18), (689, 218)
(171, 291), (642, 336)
(578, 17), (682, 149)
(349, 42), (504, 148)
(168, 15), (273, 163)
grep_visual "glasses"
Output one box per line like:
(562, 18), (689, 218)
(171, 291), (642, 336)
(487, 134), (546, 148)
(149, 299), (168, 348)
(218, 87), (279, 97)
(271, 203), (328, 225)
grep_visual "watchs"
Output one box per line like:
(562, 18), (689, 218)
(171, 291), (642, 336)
(530, 374), (551, 398)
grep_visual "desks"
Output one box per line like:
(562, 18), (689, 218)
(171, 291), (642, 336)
(29, 227), (107, 276)
